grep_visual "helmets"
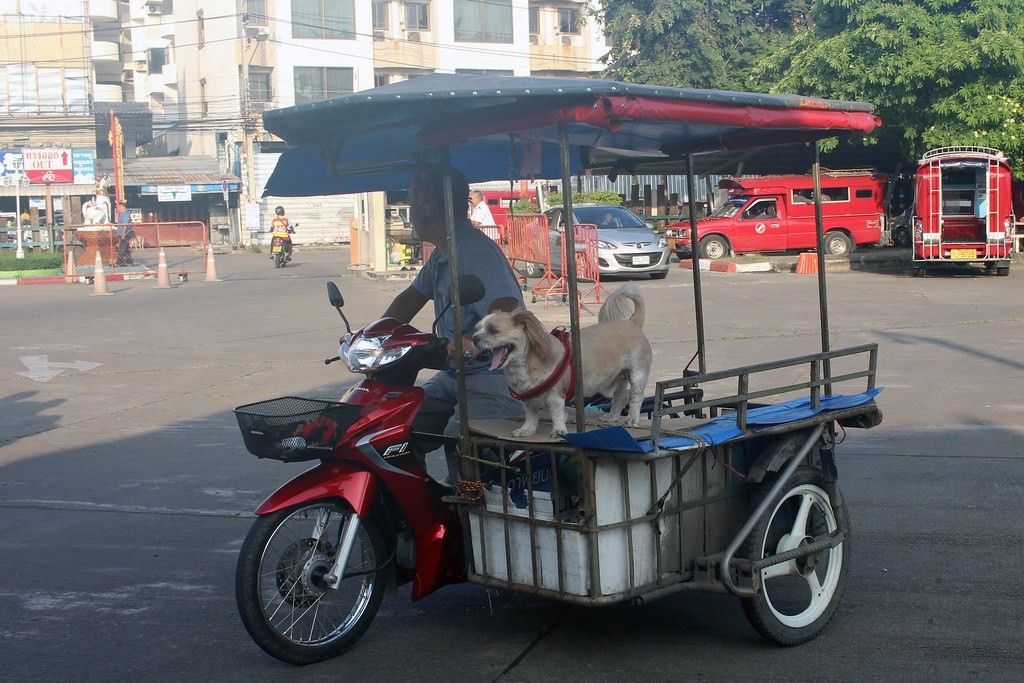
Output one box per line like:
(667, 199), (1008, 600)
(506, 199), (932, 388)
(276, 206), (284, 215)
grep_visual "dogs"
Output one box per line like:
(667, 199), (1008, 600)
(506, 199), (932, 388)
(81, 170), (112, 225)
(469, 282), (654, 437)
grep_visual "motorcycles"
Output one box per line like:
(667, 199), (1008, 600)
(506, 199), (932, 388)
(233, 281), (633, 663)
(269, 223), (298, 268)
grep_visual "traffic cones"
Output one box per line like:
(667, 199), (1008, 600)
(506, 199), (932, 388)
(88, 251), (115, 296)
(201, 244), (222, 282)
(150, 247), (176, 289)
(64, 251), (79, 283)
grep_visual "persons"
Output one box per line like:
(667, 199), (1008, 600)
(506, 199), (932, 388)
(820, 188), (832, 202)
(597, 210), (618, 229)
(557, 220), (568, 232)
(339, 166), (527, 488)
(793, 188), (816, 205)
(268, 206), (295, 261)
(467, 190), (501, 246)
(761, 204), (775, 219)
(109, 202), (134, 268)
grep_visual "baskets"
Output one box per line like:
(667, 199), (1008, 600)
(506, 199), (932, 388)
(231, 394), (365, 464)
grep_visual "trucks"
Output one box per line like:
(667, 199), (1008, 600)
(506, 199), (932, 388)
(912, 146), (1016, 276)
(665, 167), (887, 259)
(481, 187), (537, 244)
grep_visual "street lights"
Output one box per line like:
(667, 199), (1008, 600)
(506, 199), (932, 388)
(242, 31), (268, 252)
(2, 159), (30, 259)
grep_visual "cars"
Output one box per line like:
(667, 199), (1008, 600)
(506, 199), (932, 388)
(522, 202), (670, 283)
(888, 205), (913, 247)
(54, 214), (64, 225)
(27, 217), (46, 226)
(7, 227), (16, 238)
